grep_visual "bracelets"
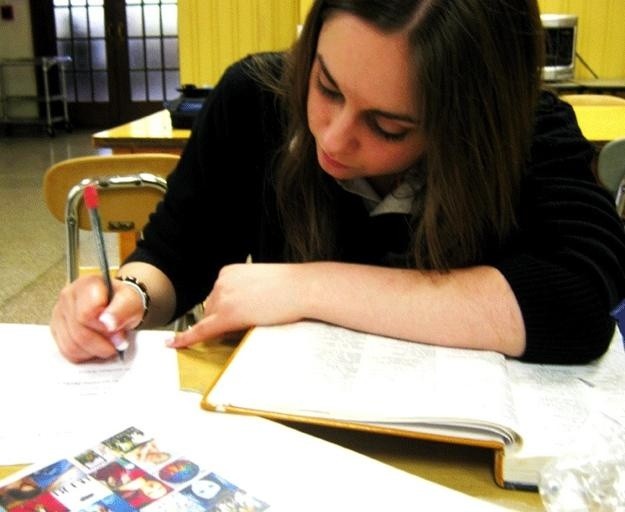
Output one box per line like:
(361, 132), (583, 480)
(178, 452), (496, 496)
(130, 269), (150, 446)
(116, 274), (151, 331)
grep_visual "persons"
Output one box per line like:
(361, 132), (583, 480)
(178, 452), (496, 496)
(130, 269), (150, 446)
(50, 0), (625, 367)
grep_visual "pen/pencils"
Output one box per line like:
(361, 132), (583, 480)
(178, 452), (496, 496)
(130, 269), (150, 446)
(83, 184), (124, 363)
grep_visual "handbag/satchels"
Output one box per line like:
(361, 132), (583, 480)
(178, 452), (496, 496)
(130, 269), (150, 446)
(164, 81), (214, 131)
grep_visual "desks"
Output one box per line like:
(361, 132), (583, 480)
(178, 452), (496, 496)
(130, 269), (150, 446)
(0, 322), (540, 512)
(94, 93), (625, 268)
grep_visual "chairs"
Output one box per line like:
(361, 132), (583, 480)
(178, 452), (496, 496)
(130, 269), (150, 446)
(43, 154), (182, 285)
(597, 135), (624, 218)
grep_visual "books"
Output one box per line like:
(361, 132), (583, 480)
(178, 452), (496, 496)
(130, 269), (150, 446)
(199, 320), (625, 495)
